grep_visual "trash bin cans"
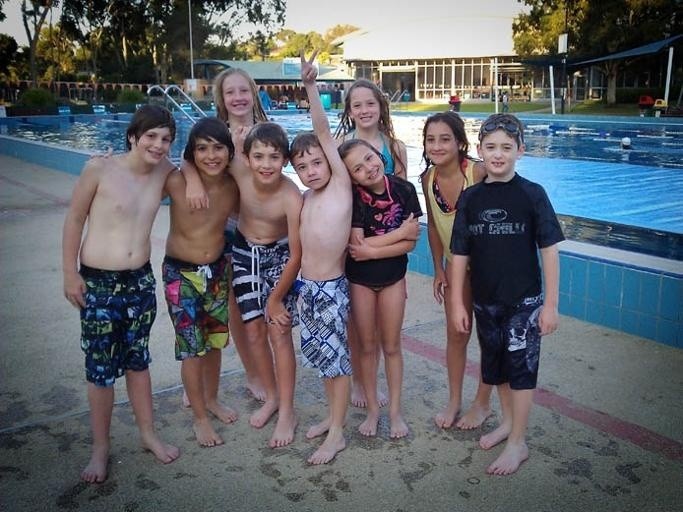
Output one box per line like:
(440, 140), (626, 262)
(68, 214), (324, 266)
(449, 95), (461, 112)
(654, 99), (668, 117)
(320, 91), (331, 110)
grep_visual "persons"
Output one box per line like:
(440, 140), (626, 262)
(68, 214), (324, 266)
(473, 86), (510, 113)
(449, 114), (566, 475)
(454, 91), (459, 102)
(621, 137), (634, 149)
(63, 48), (422, 484)
(520, 82), (532, 96)
(417, 111), (495, 430)
